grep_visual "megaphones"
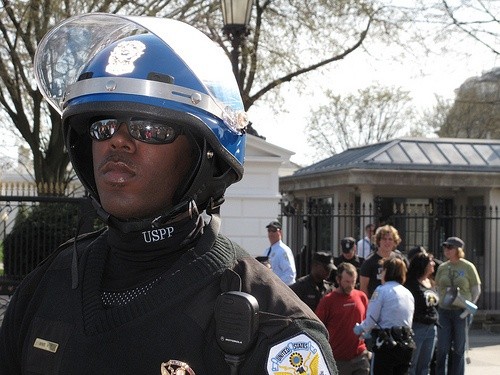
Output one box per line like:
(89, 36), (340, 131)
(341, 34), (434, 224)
(443, 286), (478, 319)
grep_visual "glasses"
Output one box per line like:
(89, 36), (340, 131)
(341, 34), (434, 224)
(442, 244), (455, 250)
(88, 118), (203, 145)
(268, 228), (280, 232)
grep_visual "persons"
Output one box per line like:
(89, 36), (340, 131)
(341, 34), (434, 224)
(0, 12), (337, 375)
(252, 219), (483, 375)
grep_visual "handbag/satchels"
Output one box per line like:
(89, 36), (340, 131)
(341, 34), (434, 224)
(364, 326), (410, 353)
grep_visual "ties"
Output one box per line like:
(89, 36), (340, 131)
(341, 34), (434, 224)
(267, 247), (271, 256)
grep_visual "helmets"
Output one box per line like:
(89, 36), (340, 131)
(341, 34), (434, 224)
(34, 13), (247, 183)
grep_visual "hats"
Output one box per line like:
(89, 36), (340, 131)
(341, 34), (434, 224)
(442, 237), (464, 247)
(266, 222), (281, 230)
(341, 237), (356, 253)
(311, 252), (338, 270)
